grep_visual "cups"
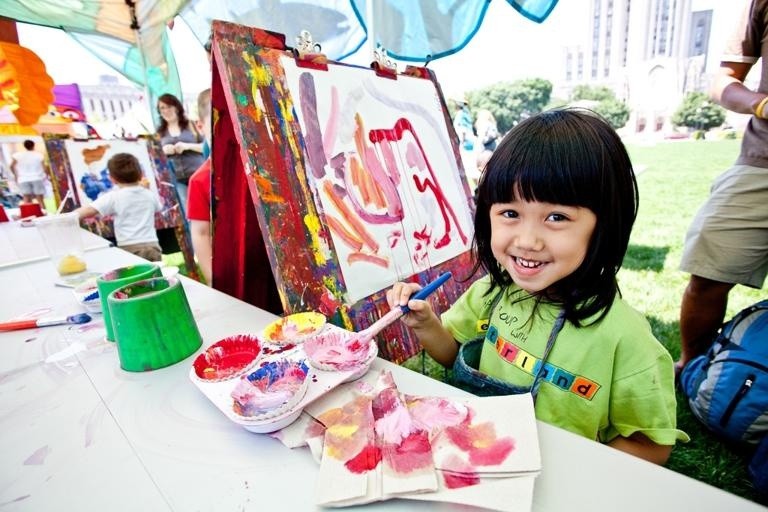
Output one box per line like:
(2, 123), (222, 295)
(32, 211), (87, 278)
(0, 204), (44, 223)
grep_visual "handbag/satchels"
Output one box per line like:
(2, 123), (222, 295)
(679, 300), (768, 447)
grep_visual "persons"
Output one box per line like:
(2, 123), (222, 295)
(157, 94), (204, 256)
(186, 89), (212, 288)
(386, 106), (690, 466)
(674, 1), (768, 386)
(10, 141), (47, 209)
(72, 153), (162, 261)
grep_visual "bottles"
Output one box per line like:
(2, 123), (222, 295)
(95, 264), (165, 343)
(106, 275), (204, 374)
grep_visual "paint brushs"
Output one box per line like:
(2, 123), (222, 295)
(348, 271), (452, 352)
(0, 314), (91, 332)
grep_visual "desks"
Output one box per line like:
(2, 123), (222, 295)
(0, 217), (115, 276)
(0, 244), (767, 512)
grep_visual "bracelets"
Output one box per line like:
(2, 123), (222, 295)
(755, 97), (768, 118)
